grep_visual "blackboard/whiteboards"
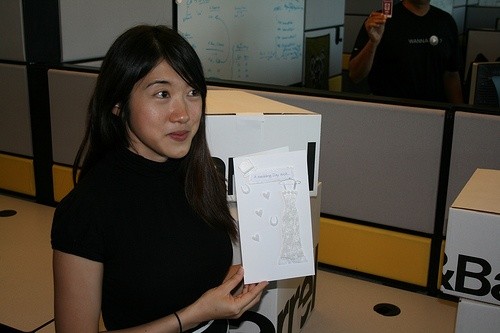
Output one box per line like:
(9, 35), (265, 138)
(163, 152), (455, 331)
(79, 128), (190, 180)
(173, 0), (306, 86)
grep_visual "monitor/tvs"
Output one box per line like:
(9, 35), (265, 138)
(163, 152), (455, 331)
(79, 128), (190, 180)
(469, 62), (500, 109)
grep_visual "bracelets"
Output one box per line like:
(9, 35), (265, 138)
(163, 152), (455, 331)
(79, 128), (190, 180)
(174, 311), (183, 333)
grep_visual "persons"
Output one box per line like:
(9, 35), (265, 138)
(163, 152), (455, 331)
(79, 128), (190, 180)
(50, 23), (268, 333)
(349, 0), (462, 104)
